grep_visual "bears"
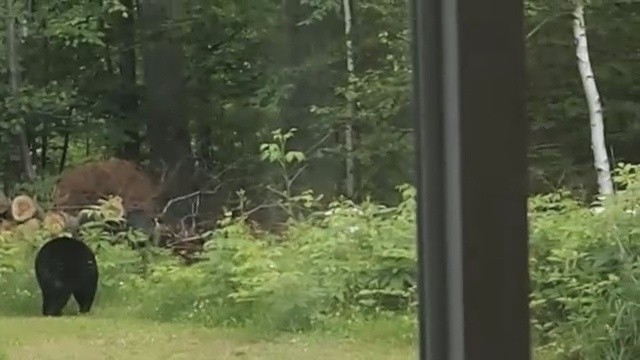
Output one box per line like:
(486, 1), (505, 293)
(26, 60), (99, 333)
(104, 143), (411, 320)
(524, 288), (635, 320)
(35, 237), (98, 316)
